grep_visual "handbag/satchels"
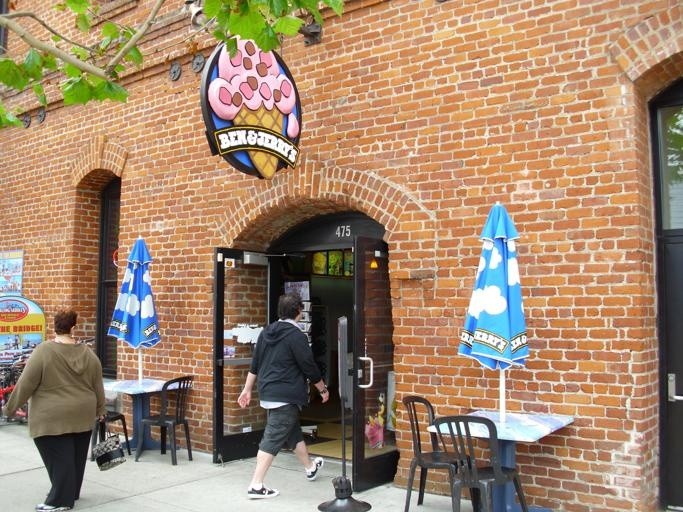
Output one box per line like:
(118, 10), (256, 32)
(92, 413), (126, 471)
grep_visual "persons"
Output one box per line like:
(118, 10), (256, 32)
(0, 309), (107, 511)
(232, 290), (330, 499)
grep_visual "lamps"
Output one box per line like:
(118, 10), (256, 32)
(188, 3), (207, 28)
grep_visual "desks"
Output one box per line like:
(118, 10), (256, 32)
(101, 378), (191, 462)
(427, 410), (574, 512)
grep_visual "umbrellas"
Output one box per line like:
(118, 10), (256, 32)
(455, 200), (531, 427)
(106, 234), (161, 382)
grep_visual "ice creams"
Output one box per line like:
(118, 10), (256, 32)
(206, 35), (298, 181)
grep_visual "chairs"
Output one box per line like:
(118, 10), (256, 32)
(402, 396), (470, 512)
(90, 411), (131, 461)
(433, 415), (530, 511)
(134, 375), (194, 465)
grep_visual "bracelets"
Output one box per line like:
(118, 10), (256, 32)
(317, 385), (327, 395)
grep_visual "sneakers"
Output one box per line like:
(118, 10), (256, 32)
(34, 502), (73, 512)
(307, 457), (324, 481)
(247, 485), (281, 499)
